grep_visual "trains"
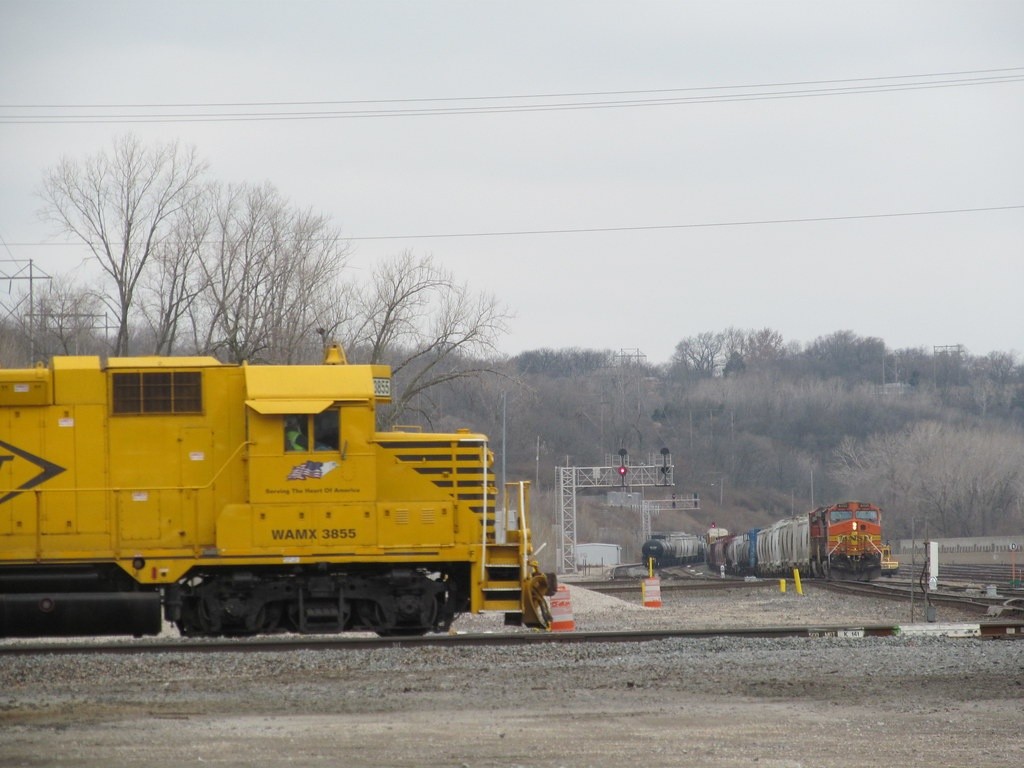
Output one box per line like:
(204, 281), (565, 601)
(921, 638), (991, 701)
(705, 502), (883, 583)
(641, 531), (708, 571)
(0, 342), (557, 636)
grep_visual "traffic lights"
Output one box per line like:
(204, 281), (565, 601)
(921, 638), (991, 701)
(660, 447), (670, 485)
(618, 448), (628, 485)
